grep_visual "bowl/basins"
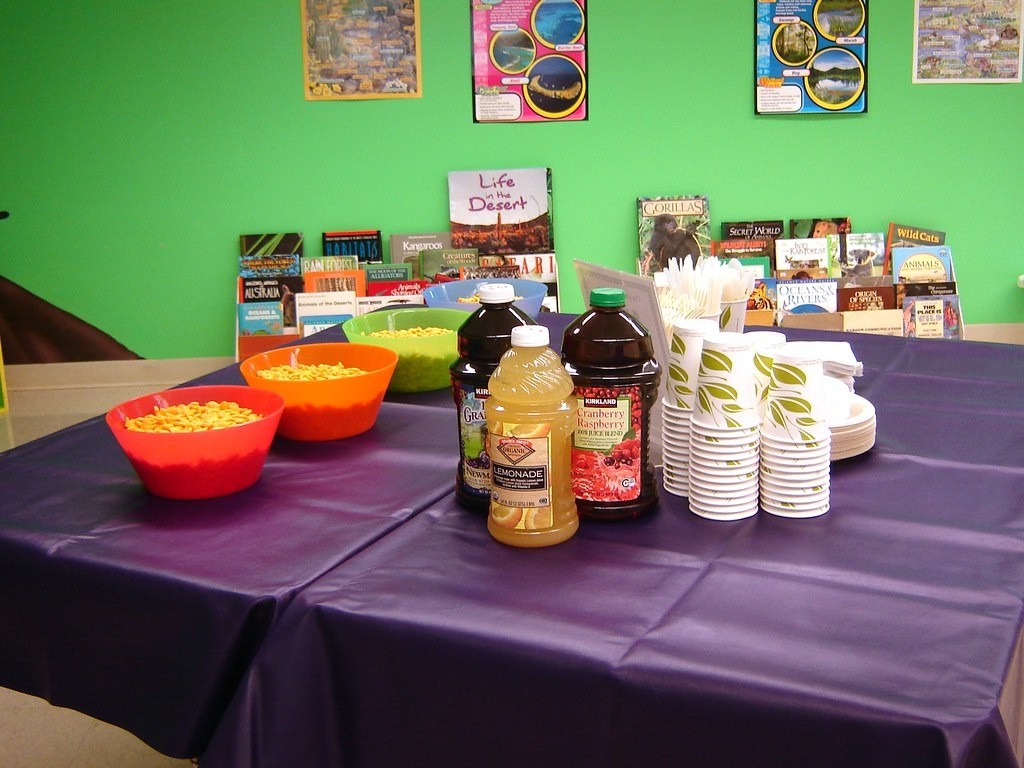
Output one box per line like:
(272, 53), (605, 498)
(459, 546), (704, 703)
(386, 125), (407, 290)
(105, 385), (285, 500)
(421, 278), (548, 320)
(341, 307), (474, 392)
(239, 343), (399, 442)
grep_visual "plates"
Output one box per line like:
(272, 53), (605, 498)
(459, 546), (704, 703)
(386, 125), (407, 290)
(826, 393), (876, 461)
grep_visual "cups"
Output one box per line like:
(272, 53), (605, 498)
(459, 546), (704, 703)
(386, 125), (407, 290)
(661, 298), (831, 522)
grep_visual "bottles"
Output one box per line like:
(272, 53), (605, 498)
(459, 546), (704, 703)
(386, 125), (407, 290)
(483, 325), (578, 548)
(560, 287), (660, 524)
(447, 283), (539, 513)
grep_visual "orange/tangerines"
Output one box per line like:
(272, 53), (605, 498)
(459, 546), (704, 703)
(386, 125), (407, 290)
(492, 506), (522, 529)
(525, 507), (551, 530)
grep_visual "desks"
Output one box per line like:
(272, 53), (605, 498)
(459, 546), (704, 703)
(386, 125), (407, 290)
(0, 325), (1024, 768)
